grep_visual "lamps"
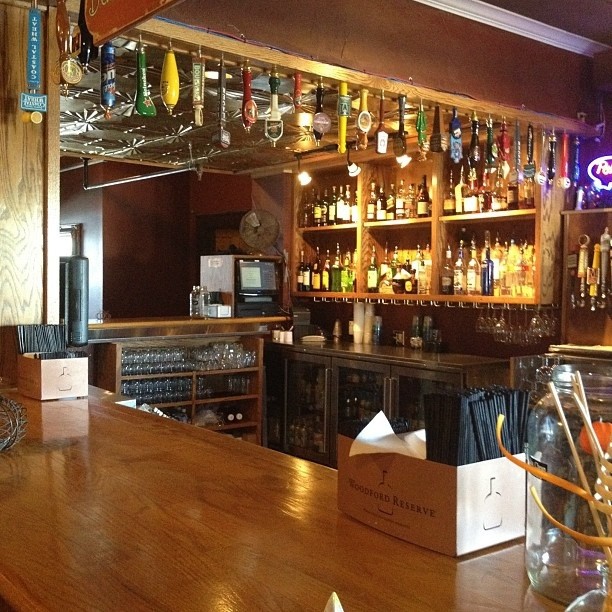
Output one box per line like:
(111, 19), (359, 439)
(396, 133), (412, 169)
(347, 142), (362, 177)
(293, 152), (312, 188)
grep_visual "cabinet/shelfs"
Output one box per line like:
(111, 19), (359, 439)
(268, 334), (513, 471)
(93, 315), (299, 449)
(288, 133), (555, 307)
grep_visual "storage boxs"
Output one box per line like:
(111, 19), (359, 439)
(17, 352), (89, 400)
(336, 419), (542, 558)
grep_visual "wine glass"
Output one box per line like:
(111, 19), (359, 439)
(474, 306), (559, 349)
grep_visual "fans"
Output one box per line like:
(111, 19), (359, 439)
(239, 210), (280, 254)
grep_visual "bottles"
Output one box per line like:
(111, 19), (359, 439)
(372, 316), (383, 346)
(390, 246), (400, 277)
(376, 185), (387, 221)
(328, 186), (337, 227)
(520, 172), (534, 208)
(297, 251), (305, 292)
(189, 285), (204, 319)
(352, 248), (357, 292)
(494, 237), (535, 298)
(526, 364), (612, 604)
(439, 243), (455, 295)
(311, 247), (323, 291)
(367, 246), (378, 291)
(454, 165), (468, 216)
(331, 243), (341, 291)
(165, 406), (187, 423)
(443, 170), (456, 216)
(302, 254), (312, 292)
(351, 190), (358, 223)
(403, 259), (415, 293)
(367, 183), (376, 221)
(416, 174), (430, 217)
(466, 233), (481, 295)
(335, 185), (344, 225)
(322, 250), (331, 291)
(417, 260), (429, 294)
(395, 179), (405, 220)
(479, 168), (493, 212)
(302, 192), (311, 227)
(320, 189), (328, 227)
(312, 191), (321, 228)
(425, 243), (431, 259)
(416, 245), (424, 261)
(480, 239), (494, 296)
(378, 245), (389, 278)
(463, 163), (479, 214)
(342, 185), (352, 224)
(404, 183), (417, 219)
(353, 302), (363, 343)
(454, 240), (467, 295)
(364, 303), (375, 344)
(384, 184), (396, 221)
(309, 188), (315, 226)
(508, 172), (516, 210)
(491, 162), (507, 212)
(199, 286), (209, 318)
(341, 248), (352, 292)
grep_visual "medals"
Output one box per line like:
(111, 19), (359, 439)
(19, 6), (48, 125)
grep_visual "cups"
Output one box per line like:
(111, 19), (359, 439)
(120, 342), (259, 375)
(224, 375), (251, 394)
(120, 377), (214, 404)
(284, 331), (293, 343)
(279, 331), (284, 343)
(272, 330), (279, 343)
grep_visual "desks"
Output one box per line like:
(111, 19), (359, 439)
(0, 379), (612, 612)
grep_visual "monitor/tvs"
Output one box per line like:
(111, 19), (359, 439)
(236, 259), (282, 300)
